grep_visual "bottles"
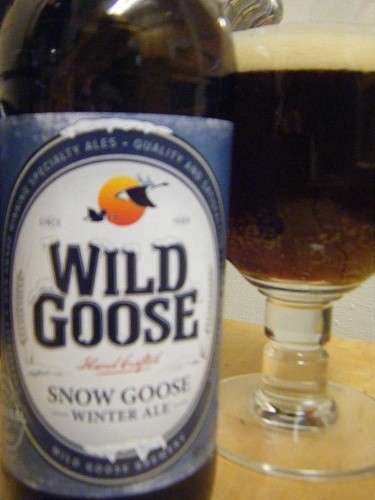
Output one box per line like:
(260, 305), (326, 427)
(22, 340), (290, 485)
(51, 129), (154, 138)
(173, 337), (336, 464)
(0, 0), (238, 500)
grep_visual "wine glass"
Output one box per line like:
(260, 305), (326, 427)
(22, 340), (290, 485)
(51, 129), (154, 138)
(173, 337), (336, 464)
(212, 21), (374, 484)
